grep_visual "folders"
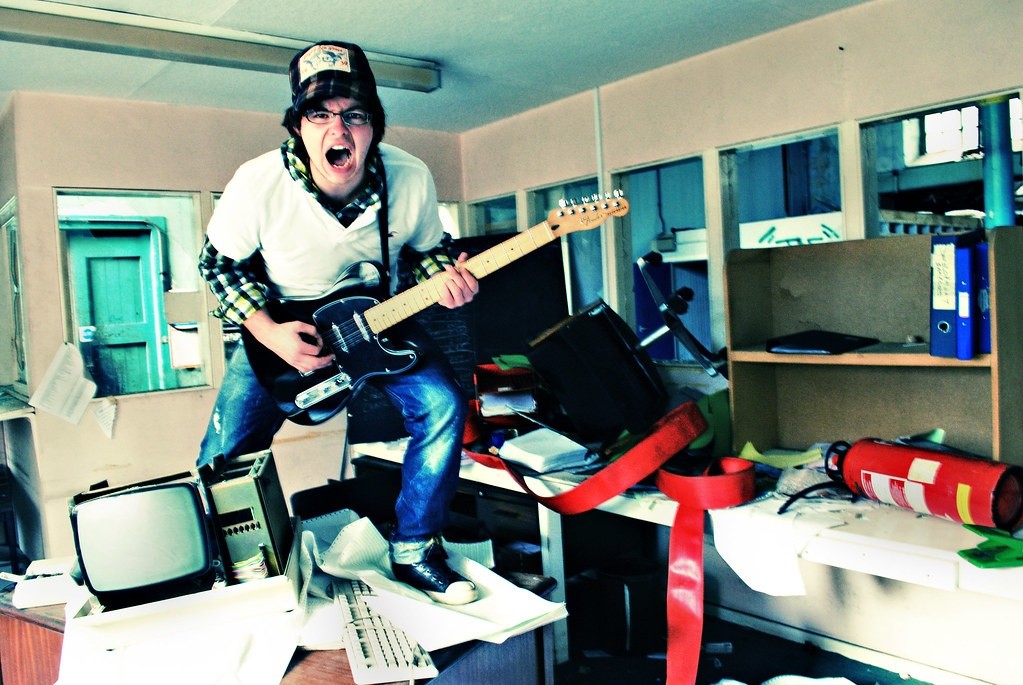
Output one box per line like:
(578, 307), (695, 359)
(930, 228), (992, 361)
(765, 328), (880, 355)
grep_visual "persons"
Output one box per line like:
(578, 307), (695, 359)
(197, 40), (478, 606)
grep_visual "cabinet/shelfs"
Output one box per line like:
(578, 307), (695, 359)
(720, 226), (1023, 466)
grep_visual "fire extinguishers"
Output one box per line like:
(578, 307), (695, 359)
(778, 435), (1022, 530)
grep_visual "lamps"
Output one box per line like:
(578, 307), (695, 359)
(0, 0), (443, 94)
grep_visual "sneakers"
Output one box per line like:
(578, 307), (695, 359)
(210, 556), (228, 588)
(390, 540), (480, 605)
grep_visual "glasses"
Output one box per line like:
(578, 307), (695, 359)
(301, 108), (372, 126)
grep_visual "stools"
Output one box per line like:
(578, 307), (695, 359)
(596, 557), (663, 657)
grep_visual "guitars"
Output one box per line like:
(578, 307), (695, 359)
(239, 186), (631, 428)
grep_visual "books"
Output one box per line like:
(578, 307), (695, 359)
(765, 330), (880, 355)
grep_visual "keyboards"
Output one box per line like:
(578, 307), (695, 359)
(332, 574), (440, 685)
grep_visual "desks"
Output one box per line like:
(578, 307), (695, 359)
(0, 570), (558, 685)
(350, 405), (1023, 685)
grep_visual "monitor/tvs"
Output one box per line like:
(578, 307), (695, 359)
(68, 468), (220, 611)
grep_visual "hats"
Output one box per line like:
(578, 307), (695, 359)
(288, 41), (376, 111)
